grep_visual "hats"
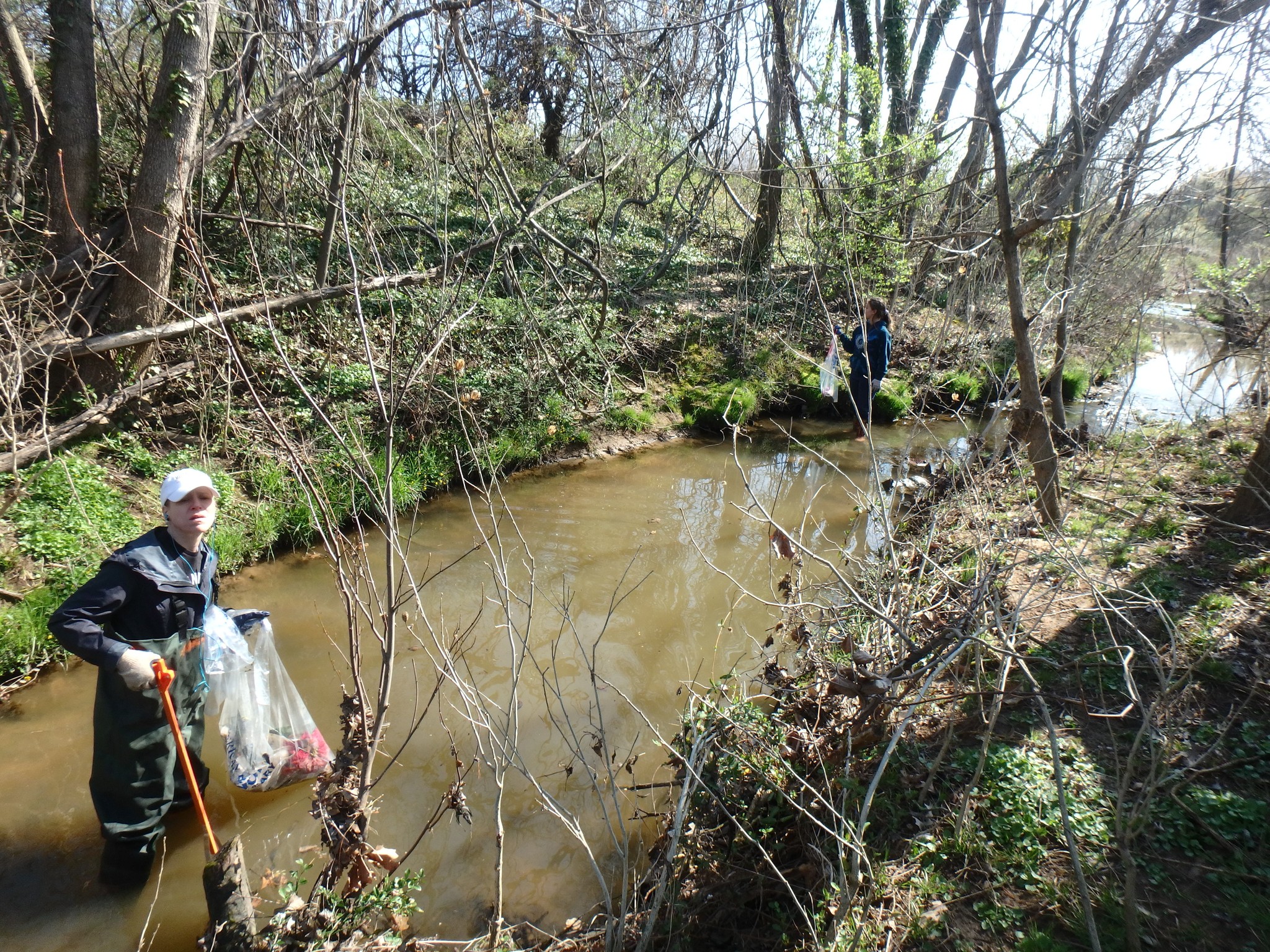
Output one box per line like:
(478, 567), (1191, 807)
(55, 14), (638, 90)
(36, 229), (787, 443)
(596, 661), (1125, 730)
(161, 468), (220, 508)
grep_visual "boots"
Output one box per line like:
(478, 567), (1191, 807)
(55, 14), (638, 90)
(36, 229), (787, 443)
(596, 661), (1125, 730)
(99, 837), (153, 905)
(851, 417), (868, 442)
(167, 771), (210, 817)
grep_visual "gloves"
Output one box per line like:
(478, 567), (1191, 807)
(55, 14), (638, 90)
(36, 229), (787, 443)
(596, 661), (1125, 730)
(871, 378), (881, 392)
(225, 605), (271, 635)
(834, 325), (843, 336)
(116, 651), (161, 690)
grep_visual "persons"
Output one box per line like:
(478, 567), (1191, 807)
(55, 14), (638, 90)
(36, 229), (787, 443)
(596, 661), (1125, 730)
(833, 299), (892, 437)
(47, 468), (270, 894)
